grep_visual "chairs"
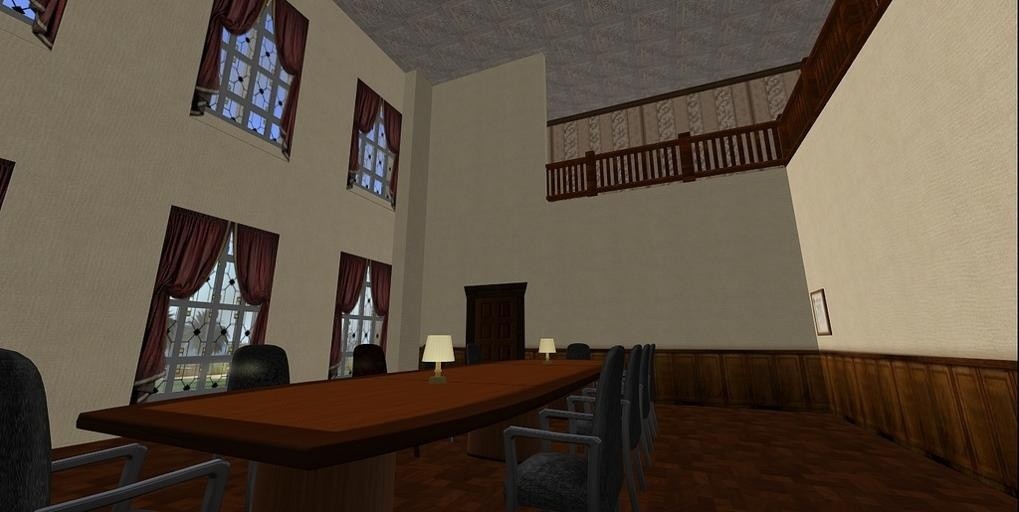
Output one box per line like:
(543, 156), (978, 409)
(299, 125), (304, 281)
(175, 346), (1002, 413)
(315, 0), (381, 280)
(350, 344), (390, 377)
(0, 345), (231, 511)
(214, 343), (290, 510)
(501, 342), (662, 512)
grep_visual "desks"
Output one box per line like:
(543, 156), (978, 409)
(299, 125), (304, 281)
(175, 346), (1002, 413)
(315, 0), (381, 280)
(76, 359), (612, 512)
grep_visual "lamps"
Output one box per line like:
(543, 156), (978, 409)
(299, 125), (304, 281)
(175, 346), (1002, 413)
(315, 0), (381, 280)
(420, 334), (459, 383)
(538, 338), (557, 364)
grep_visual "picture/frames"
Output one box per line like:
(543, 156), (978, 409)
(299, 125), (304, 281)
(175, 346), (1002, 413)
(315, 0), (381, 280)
(809, 287), (833, 336)
